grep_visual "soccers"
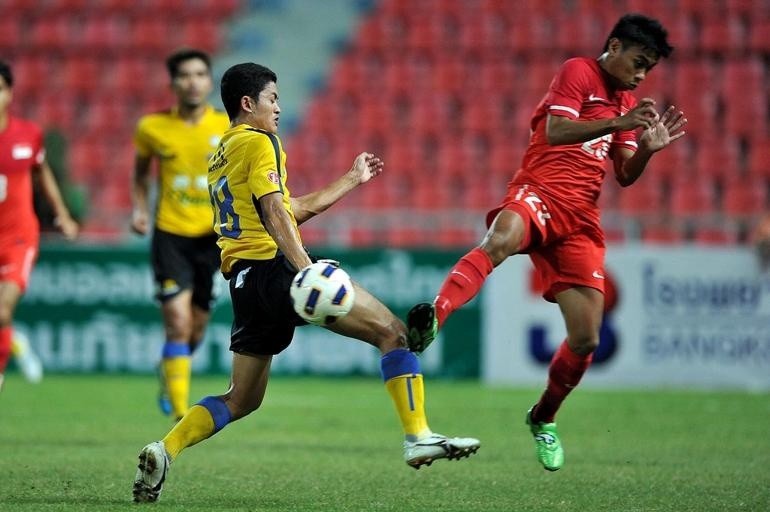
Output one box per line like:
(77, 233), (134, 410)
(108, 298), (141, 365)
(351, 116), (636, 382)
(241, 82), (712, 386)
(290, 263), (354, 327)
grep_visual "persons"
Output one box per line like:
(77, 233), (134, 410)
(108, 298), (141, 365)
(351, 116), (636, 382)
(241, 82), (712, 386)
(130, 49), (231, 428)
(0, 63), (78, 391)
(407, 14), (688, 472)
(133, 62), (480, 503)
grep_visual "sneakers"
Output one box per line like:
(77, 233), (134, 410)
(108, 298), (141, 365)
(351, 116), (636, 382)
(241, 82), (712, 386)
(157, 366), (174, 416)
(9, 330), (42, 385)
(526, 405), (564, 471)
(403, 434), (480, 469)
(405, 302), (437, 357)
(134, 440), (169, 502)
